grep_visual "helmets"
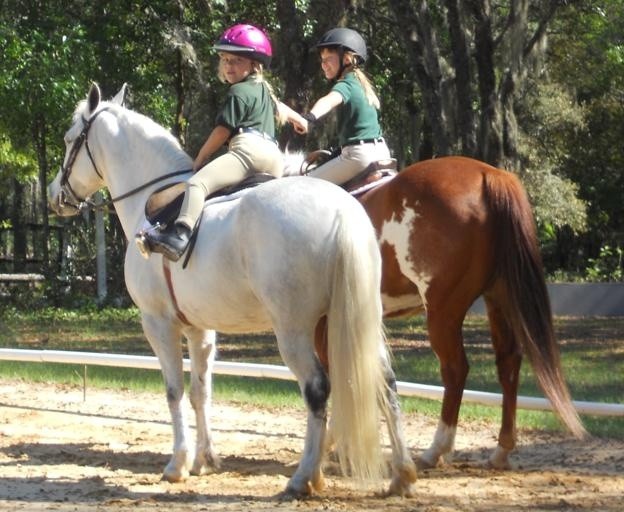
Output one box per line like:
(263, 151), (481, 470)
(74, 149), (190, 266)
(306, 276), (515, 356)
(315, 28), (368, 64)
(208, 24), (273, 70)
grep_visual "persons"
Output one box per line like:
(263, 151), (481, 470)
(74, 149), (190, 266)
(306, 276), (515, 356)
(302, 26), (393, 186)
(144, 22), (311, 264)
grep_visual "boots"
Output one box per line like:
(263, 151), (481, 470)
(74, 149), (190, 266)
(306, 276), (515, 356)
(142, 222), (194, 262)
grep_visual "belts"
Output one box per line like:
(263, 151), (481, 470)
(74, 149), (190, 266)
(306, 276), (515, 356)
(228, 127), (283, 153)
(342, 139), (382, 147)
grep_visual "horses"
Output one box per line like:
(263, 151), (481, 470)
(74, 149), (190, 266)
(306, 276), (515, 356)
(278, 136), (590, 470)
(48, 79), (418, 501)
